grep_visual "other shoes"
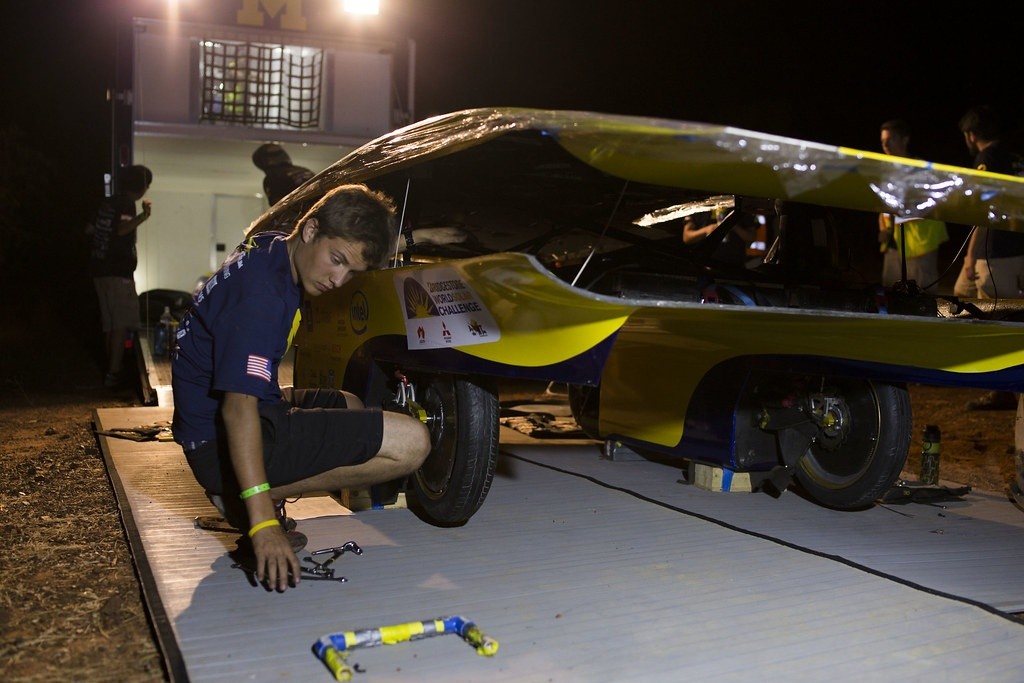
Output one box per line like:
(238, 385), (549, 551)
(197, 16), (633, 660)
(205, 491), (308, 554)
(104, 370), (130, 387)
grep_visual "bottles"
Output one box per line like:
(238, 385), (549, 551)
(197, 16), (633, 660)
(919, 424), (941, 485)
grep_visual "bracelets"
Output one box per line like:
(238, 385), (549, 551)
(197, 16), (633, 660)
(248, 519), (280, 537)
(239, 483), (270, 500)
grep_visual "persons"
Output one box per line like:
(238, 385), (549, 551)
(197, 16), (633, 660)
(83, 165), (152, 388)
(878, 120), (951, 288)
(682, 200), (758, 255)
(954, 103), (1024, 412)
(252, 144), (316, 205)
(170, 184), (432, 592)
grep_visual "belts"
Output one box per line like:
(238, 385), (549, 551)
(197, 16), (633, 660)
(181, 440), (207, 452)
